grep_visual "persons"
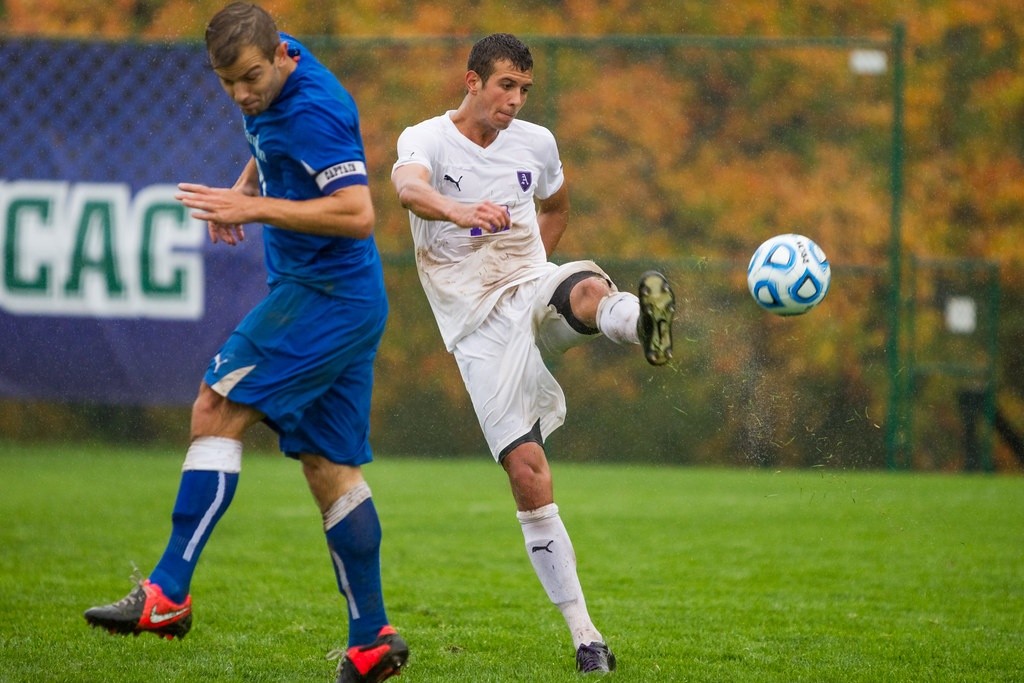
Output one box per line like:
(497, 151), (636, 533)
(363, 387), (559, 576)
(391, 33), (676, 673)
(84, 0), (411, 683)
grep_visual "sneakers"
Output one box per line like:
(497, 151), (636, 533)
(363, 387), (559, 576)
(575, 641), (616, 676)
(82, 561), (192, 640)
(635, 271), (674, 365)
(325, 624), (409, 682)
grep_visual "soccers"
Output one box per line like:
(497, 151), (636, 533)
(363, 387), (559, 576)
(746, 233), (831, 317)
